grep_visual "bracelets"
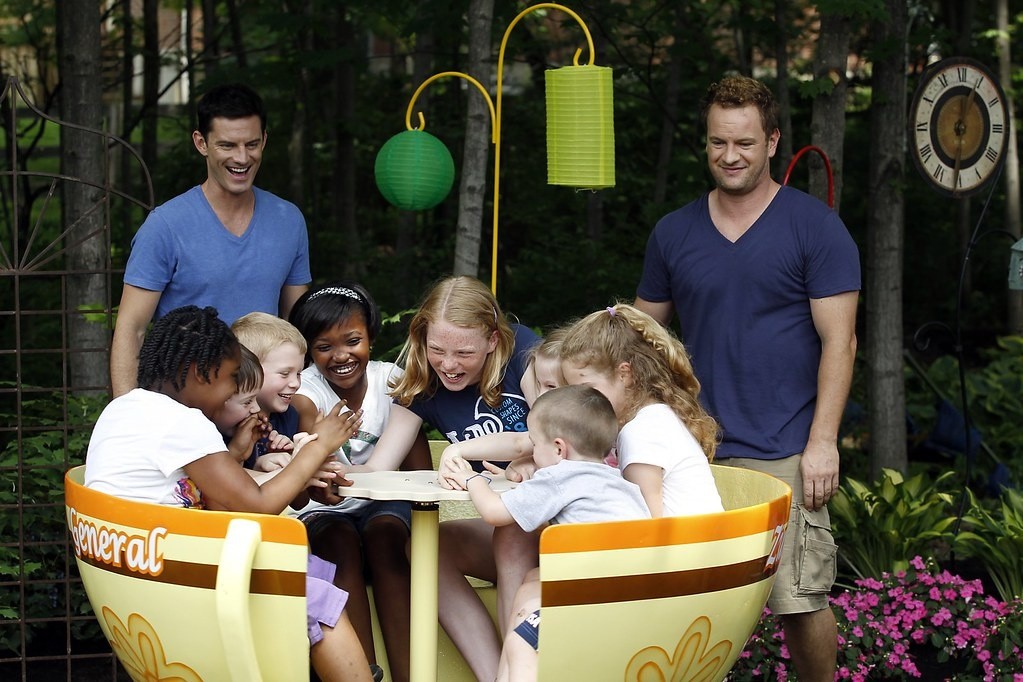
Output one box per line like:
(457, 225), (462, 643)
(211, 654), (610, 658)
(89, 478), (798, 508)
(466, 473), (492, 489)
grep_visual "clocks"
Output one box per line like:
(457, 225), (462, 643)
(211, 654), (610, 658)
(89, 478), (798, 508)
(907, 55), (1010, 200)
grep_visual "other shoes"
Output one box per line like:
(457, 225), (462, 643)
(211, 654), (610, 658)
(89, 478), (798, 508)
(310, 664), (383, 682)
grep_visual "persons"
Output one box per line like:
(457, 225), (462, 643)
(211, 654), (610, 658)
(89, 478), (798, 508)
(214, 347), (385, 682)
(633, 75), (861, 682)
(110, 84), (312, 402)
(228, 276), (727, 682)
(83, 303), (375, 682)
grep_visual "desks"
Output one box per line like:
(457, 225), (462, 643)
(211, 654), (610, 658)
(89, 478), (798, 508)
(338, 469), (524, 682)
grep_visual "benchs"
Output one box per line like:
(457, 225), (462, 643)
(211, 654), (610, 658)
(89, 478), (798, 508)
(64, 440), (792, 682)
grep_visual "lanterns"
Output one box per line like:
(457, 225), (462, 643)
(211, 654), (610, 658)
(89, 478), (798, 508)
(375, 129), (456, 210)
(544, 66), (614, 186)
